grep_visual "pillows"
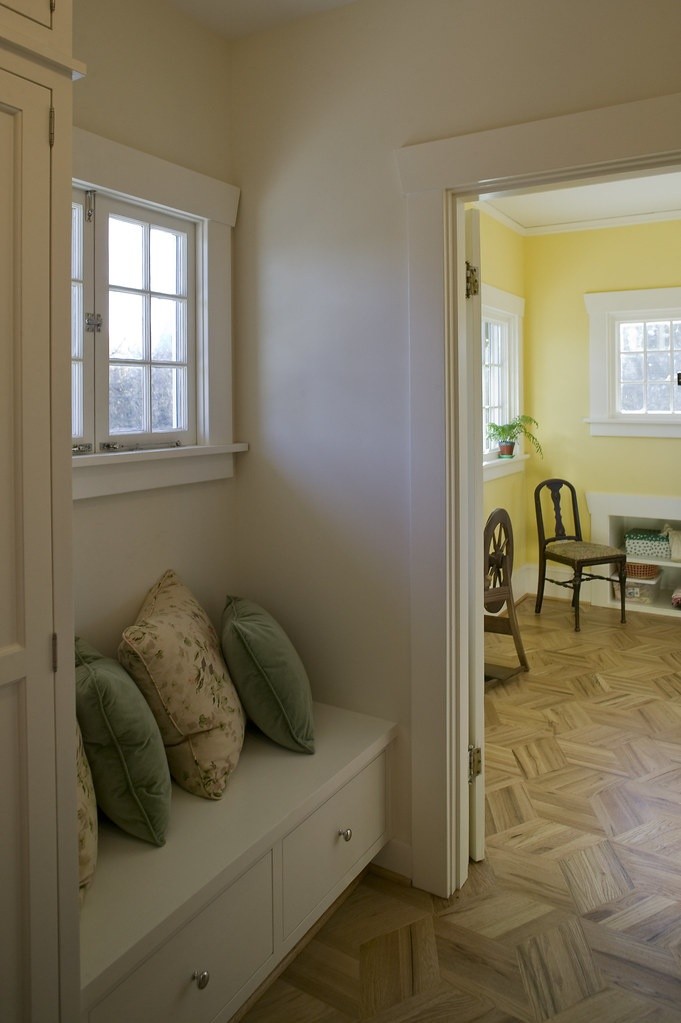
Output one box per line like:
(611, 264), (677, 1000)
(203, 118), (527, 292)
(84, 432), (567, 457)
(221, 593), (316, 756)
(75, 634), (172, 846)
(75, 717), (97, 912)
(119, 568), (246, 801)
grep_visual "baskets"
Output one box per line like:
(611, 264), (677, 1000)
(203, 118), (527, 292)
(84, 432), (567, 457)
(617, 561), (661, 579)
(624, 528), (671, 559)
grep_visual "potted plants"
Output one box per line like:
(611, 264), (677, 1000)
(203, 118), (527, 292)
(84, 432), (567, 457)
(483, 415), (544, 459)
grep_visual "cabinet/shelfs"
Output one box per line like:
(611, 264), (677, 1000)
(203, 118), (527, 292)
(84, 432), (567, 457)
(86, 847), (273, 1023)
(611, 555), (681, 617)
(283, 741), (392, 969)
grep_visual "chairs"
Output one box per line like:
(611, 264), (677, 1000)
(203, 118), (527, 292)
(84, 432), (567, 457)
(534, 479), (628, 632)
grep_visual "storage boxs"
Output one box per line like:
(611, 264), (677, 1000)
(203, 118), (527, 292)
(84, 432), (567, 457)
(624, 528), (670, 559)
(611, 569), (665, 605)
